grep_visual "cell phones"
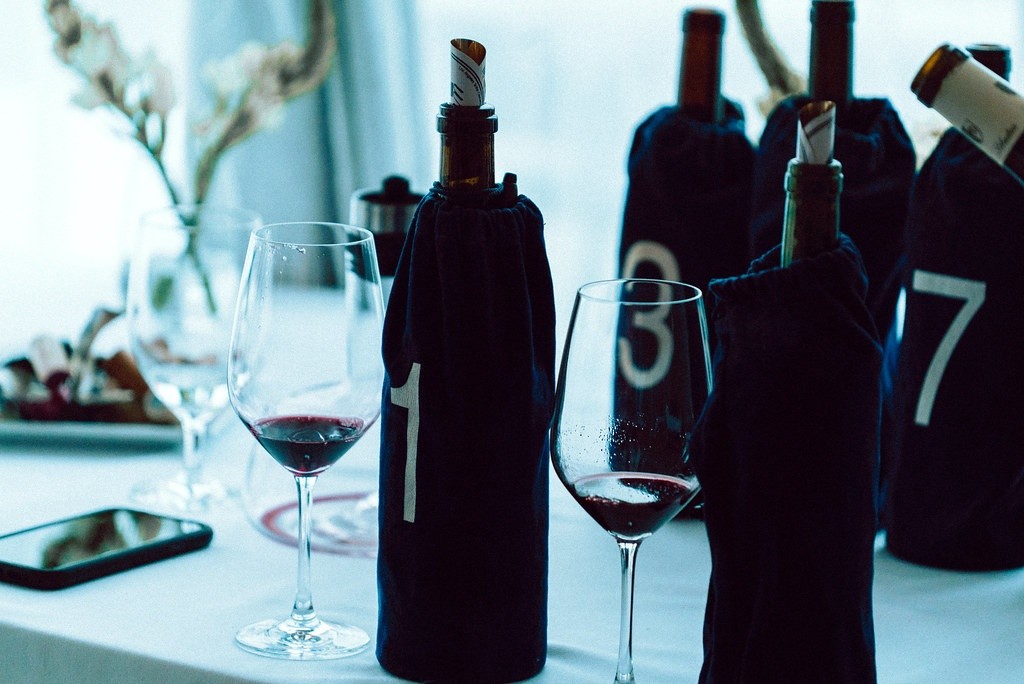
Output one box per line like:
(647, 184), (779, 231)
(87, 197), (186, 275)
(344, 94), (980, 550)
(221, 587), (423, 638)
(0, 506), (214, 590)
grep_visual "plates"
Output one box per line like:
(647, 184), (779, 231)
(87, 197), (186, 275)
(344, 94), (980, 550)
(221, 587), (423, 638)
(6, 411), (207, 448)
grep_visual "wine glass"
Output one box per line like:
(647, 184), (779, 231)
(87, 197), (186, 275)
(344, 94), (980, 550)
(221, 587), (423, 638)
(554, 275), (709, 681)
(124, 199), (271, 523)
(226, 218), (383, 660)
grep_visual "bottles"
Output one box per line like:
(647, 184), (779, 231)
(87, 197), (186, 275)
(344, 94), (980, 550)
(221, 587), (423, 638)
(376, 36), (551, 682)
(606, 0), (1021, 681)
